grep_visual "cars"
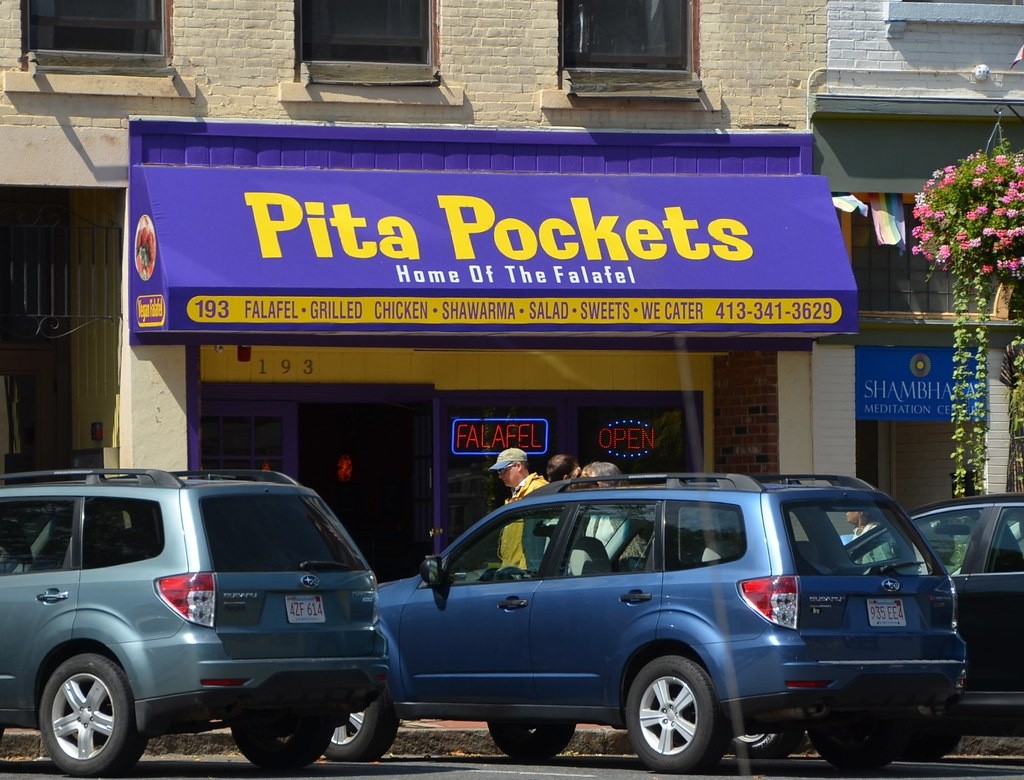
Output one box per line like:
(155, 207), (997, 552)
(726, 491), (1024, 760)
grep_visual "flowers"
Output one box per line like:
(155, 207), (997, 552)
(907, 107), (1024, 499)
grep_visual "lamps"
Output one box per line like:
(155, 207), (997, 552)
(973, 63), (991, 82)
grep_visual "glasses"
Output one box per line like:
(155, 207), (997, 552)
(497, 462), (517, 474)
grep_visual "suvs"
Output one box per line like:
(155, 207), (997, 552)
(1, 465), (390, 778)
(317, 471), (970, 776)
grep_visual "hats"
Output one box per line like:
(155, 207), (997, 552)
(488, 447), (529, 473)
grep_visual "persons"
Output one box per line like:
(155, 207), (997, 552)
(485, 446), (549, 581)
(543, 450), (581, 552)
(569, 458), (648, 576)
(844, 510), (897, 574)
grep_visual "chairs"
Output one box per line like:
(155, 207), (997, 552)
(570, 536), (613, 575)
(920, 528), (957, 577)
